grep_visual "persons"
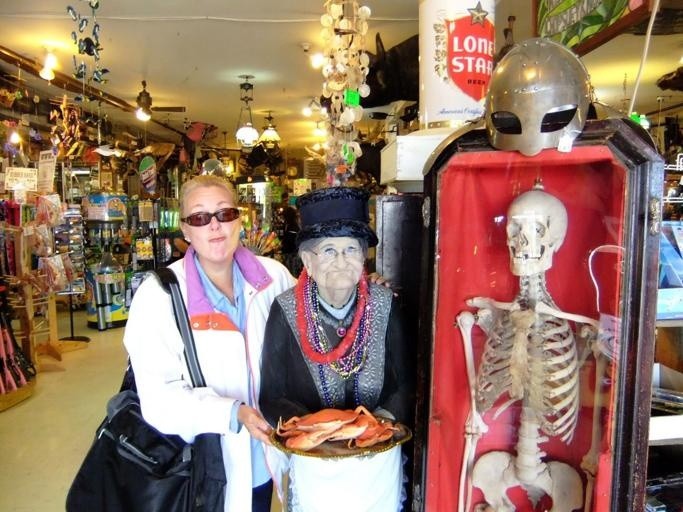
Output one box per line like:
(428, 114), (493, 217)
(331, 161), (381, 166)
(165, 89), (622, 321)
(123, 174), (398, 512)
(258, 187), (419, 512)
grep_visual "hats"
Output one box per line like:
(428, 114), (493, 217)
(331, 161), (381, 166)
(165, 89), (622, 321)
(295, 187), (378, 248)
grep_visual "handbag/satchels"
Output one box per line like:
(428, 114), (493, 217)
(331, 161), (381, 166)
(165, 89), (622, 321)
(66, 268), (226, 512)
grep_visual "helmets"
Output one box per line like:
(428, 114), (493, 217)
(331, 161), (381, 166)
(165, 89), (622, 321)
(484, 37), (592, 156)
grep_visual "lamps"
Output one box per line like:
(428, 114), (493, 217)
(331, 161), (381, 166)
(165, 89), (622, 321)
(235, 75), (280, 150)
(136, 80), (154, 121)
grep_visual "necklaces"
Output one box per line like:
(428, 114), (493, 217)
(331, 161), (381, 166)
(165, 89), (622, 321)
(295, 266), (373, 409)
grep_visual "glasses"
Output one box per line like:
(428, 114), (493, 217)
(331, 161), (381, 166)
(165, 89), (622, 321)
(181, 208), (240, 227)
(308, 245), (365, 258)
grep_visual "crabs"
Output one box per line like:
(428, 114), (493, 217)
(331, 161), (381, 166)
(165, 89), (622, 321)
(276, 405), (404, 452)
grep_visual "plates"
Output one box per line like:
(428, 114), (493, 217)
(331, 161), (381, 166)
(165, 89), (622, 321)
(269, 415), (414, 460)
